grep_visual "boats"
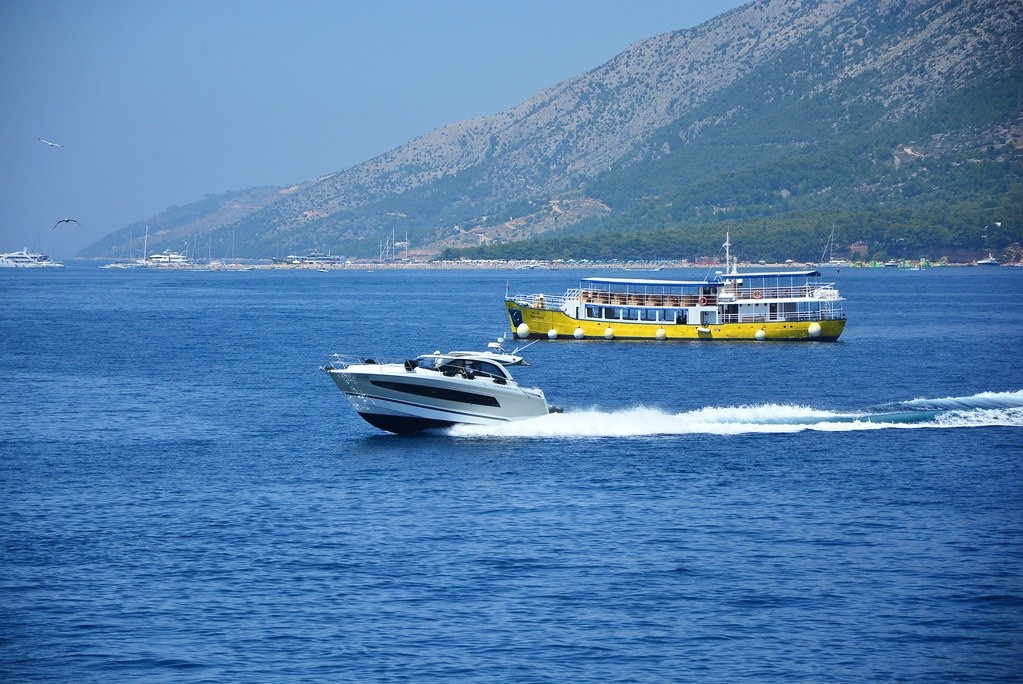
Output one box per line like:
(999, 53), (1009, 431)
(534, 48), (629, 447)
(503, 228), (850, 342)
(321, 338), (565, 436)
(289, 248), (341, 268)
(148, 249), (191, 268)
(0, 247), (66, 268)
(885, 261), (898, 268)
(976, 252), (1002, 266)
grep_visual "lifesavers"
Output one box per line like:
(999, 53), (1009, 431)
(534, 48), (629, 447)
(699, 297), (708, 305)
(754, 290), (762, 299)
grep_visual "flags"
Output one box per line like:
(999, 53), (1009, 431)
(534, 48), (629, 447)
(836, 268), (840, 273)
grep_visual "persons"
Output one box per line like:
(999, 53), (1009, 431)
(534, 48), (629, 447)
(434, 351), (443, 368)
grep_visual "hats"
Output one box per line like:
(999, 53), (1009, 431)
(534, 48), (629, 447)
(434, 350), (439, 354)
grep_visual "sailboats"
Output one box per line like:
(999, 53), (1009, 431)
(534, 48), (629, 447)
(98, 224), (149, 268)
(815, 224), (835, 269)
(183, 224), (256, 271)
